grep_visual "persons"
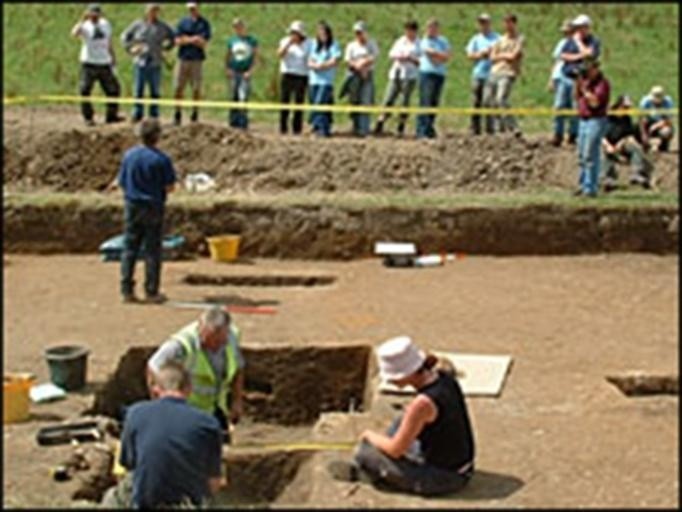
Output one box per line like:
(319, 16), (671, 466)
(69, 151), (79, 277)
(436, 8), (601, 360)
(326, 334), (474, 498)
(145, 308), (246, 444)
(70, 2), (126, 126)
(118, 2), (175, 124)
(223, 18), (259, 128)
(117, 118), (176, 303)
(170, 2), (211, 125)
(119, 362), (223, 511)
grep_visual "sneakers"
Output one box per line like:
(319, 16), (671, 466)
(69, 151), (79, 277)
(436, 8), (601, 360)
(144, 292), (170, 306)
(603, 176), (628, 191)
(628, 174), (654, 189)
(120, 293), (142, 306)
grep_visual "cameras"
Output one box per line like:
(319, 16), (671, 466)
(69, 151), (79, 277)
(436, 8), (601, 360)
(573, 65), (586, 76)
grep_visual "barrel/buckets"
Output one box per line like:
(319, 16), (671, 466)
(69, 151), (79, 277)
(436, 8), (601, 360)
(4, 373), (38, 422)
(206, 234), (240, 261)
(42, 343), (92, 388)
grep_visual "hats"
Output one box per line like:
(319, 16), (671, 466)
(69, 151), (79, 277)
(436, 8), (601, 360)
(648, 84), (667, 103)
(476, 12), (492, 24)
(572, 13), (593, 30)
(84, 3), (104, 20)
(559, 17), (577, 34)
(373, 334), (430, 384)
(287, 19), (309, 40)
(352, 21), (367, 37)
(616, 93), (634, 109)
